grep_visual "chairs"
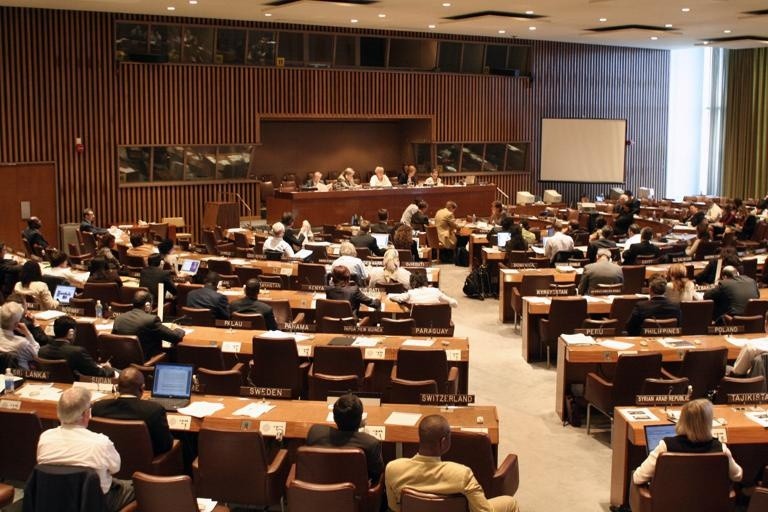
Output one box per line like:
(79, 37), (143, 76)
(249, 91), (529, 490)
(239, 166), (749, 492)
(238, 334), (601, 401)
(470, 193), (766, 512)
(1, 216), (521, 510)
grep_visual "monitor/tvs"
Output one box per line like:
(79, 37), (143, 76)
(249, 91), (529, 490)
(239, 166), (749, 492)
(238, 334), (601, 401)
(543, 190), (562, 204)
(610, 188), (624, 199)
(516, 191), (535, 206)
(639, 187), (654, 199)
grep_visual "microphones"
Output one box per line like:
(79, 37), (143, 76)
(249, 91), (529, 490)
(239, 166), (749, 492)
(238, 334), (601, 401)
(594, 318), (609, 342)
(237, 193), (244, 202)
(651, 316), (666, 339)
(715, 307), (736, 338)
(660, 385), (673, 414)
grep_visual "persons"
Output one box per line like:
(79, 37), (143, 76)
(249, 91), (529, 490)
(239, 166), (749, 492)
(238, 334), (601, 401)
(0, 164), (767, 512)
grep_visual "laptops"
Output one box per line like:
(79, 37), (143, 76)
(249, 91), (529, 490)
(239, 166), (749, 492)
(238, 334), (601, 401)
(148, 363), (194, 412)
(179, 260), (201, 276)
(53, 285), (76, 306)
(497, 232), (512, 252)
(644, 424), (678, 457)
(371, 233), (388, 250)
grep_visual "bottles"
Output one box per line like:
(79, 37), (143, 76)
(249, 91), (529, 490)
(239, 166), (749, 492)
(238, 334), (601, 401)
(350, 212), (363, 227)
(4, 367), (14, 396)
(95, 298), (103, 323)
(652, 210), (656, 221)
(472, 214), (476, 224)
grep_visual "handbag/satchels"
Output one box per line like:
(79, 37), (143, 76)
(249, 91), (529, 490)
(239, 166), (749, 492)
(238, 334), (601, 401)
(456, 247), (468, 266)
(464, 270), (498, 297)
